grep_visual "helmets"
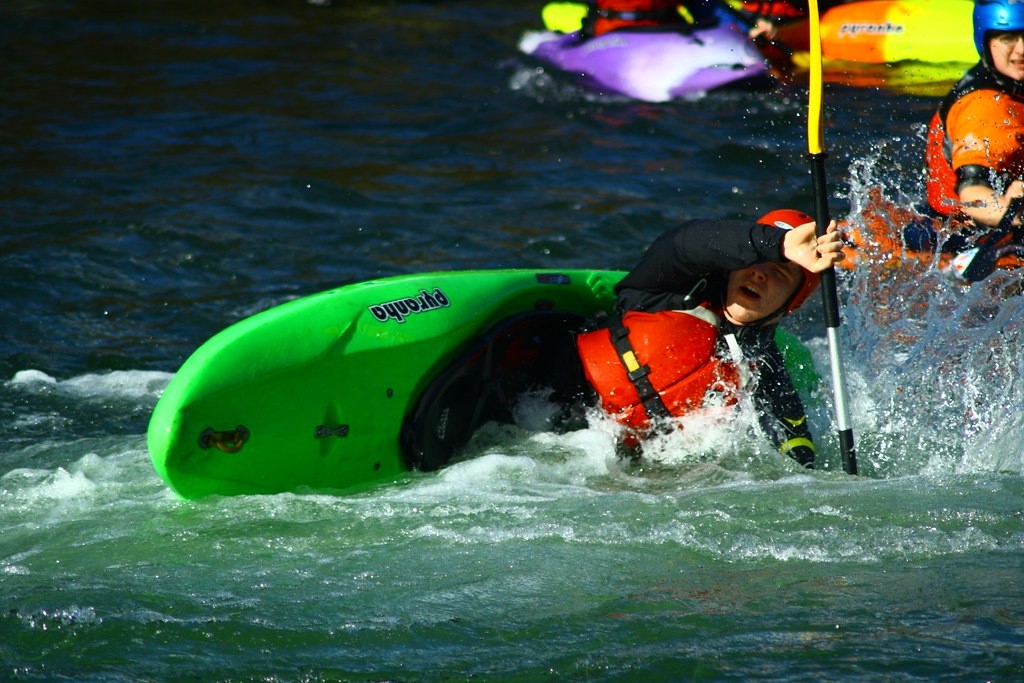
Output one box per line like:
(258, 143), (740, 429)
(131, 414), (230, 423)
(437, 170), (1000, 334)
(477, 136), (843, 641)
(973, 0), (1024, 58)
(756, 209), (820, 317)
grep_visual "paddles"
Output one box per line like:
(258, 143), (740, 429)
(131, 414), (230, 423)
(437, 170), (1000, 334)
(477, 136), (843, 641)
(948, 197), (1020, 281)
(806, 0), (858, 476)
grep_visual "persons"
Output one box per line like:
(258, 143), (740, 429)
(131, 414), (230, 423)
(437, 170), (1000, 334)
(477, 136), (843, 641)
(924, 1), (1024, 258)
(581, 0), (775, 43)
(491, 209), (847, 471)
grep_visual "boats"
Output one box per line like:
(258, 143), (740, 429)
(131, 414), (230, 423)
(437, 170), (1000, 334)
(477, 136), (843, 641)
(831, 187), (1023, 343)
(145, 265), (823, 508)
(757, 0), (982, 97)
(518, 0), (786, 105)
